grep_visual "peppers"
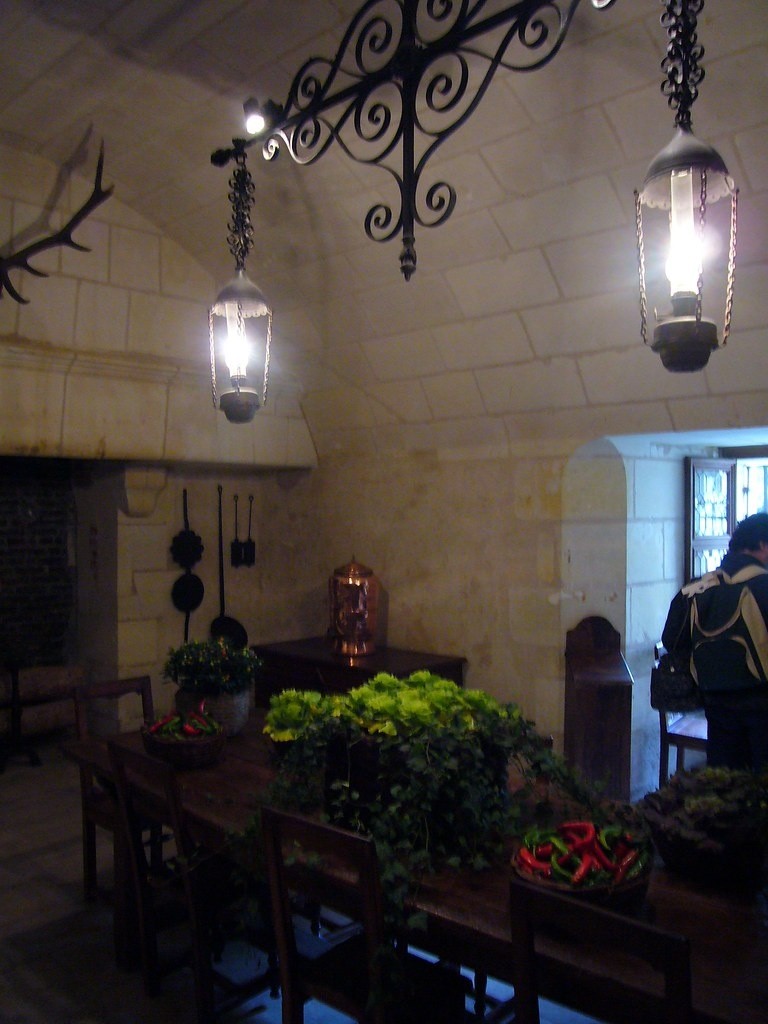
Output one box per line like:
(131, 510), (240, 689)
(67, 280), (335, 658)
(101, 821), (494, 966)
(147, 709), (222, 740)
(516, 822), (648, 885)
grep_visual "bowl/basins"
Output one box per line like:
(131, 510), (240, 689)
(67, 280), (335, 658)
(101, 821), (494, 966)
(143, 724), (226, 769)
(515, 863), (652, 919)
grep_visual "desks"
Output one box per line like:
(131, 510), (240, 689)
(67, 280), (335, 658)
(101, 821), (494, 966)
(65, 710), (768, 1024)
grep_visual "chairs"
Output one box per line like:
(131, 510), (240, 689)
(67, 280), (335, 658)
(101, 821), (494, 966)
(258, 805), (469, 1024)
(74, 674), (183, 898)
(106, 737), (271, 1024)
(508, 881), (695, 1024)
(652, 641), (707, 786)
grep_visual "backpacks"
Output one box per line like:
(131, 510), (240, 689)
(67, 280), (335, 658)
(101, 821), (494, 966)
(688, 564), (767, 687)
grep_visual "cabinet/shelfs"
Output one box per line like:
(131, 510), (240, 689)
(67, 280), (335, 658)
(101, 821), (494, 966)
(250, 636), (467, 711)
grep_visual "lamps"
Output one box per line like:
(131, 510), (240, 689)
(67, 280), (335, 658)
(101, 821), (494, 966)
(633, 0), (740, 372)
(242, 98), (282, 136)
(207, 150), (270, 425)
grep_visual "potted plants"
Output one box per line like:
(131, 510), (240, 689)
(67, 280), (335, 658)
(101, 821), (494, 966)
(639, 764), (767, 895)
(262, 668), (612, 1024)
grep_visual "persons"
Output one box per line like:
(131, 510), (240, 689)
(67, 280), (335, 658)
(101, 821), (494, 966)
(651, 512), (768, 777)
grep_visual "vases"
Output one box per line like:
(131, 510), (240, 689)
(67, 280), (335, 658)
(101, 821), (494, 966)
(175, 688), (255, 740)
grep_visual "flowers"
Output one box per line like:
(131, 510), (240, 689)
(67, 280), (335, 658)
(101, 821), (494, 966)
(159, 636), (262, 695)
